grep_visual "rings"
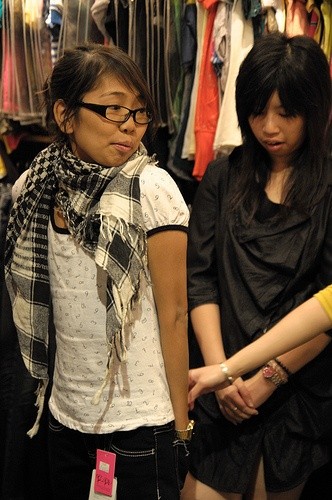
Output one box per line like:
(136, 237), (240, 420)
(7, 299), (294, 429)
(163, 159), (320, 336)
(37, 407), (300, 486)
(232, 407), (237, 413)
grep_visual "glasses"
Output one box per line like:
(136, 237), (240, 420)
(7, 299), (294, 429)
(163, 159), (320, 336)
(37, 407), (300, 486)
(80, 103), (154, 124)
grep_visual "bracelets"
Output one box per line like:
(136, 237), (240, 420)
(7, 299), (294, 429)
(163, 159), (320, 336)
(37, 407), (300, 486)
(219, 363), (235, 385)
(173, 420), (196, 432)
(262, 357), (294, 387)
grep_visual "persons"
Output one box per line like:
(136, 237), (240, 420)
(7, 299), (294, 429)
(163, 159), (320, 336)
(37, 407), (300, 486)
(187, 284), (332, 410)
(4, 42), (191, 500)
(180, 32), (332, 500)
